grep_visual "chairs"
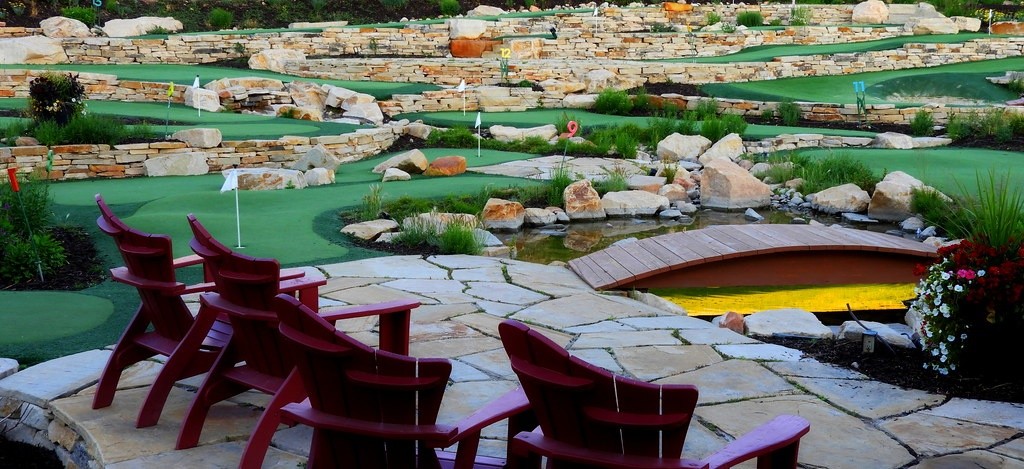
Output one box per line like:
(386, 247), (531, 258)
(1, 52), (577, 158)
(235, 293), (539, 469)
(92, 194), (306, 428)
(497, 320), (811, 469)
(137, 214), (421, 451)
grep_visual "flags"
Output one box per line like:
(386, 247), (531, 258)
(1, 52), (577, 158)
(221, 170), (237, 193)
(456, 80), (465, 93)
(989, 10), (993, 17)
(594, 8), (597, 16)
(168, 82), (173, 97)
(192, 74), (199, 89)
(474, 112), (481, 129)
